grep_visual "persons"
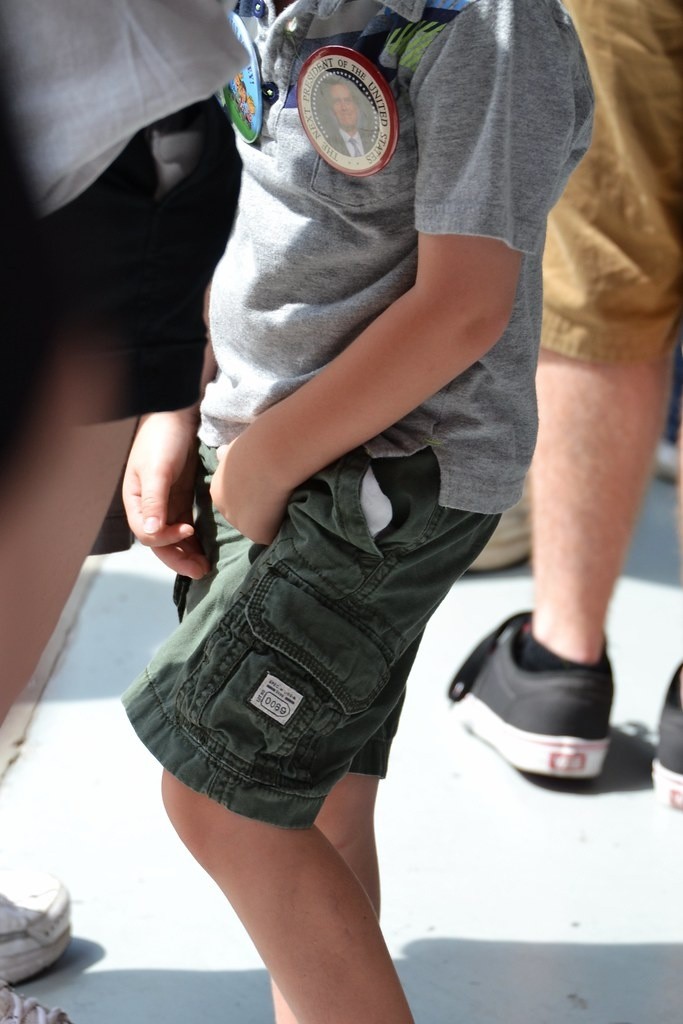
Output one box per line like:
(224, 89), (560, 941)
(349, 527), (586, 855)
(443, 3), (681, 810)
(0, 4), (248, 1024)
(120, 1), (597, 1024)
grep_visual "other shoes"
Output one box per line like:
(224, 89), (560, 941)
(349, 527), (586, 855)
(468, 494), (529, 570)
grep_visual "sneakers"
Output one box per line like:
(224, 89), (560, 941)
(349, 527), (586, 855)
(0, 872), (73, 1024)
(446, 612), (614, 778)
(651, 661), (683, 809)
